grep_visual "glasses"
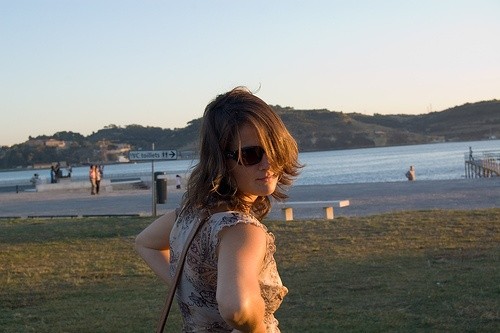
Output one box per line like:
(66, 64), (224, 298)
(227, 145), (267, 166)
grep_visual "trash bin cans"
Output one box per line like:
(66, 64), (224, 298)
(154, 172), (167, 204)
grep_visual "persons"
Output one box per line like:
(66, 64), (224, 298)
(133, 87), (307, 333)
(468, 146), (474, 162)
(406, 166), (416, 180)
(30, 161), (183, 194)
